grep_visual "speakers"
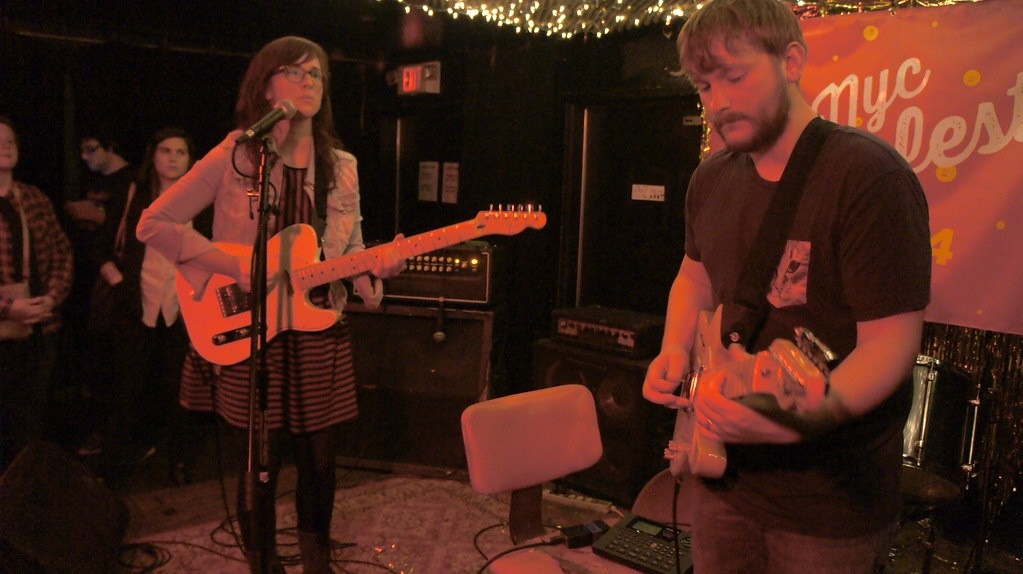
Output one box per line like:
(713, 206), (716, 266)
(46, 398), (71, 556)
(0, 440), (130, 574)
(530, 335), (682, 511)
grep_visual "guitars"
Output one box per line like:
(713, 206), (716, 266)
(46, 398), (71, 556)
(173, 202), (547, 367)
(663, 300), (834, 480)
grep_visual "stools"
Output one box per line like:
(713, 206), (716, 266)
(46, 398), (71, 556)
(461, 384), (654, 574)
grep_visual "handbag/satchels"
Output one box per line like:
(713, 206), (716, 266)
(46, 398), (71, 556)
(0, 283), (34, 340)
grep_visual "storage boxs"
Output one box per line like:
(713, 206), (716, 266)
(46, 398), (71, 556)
(335, 301), (510, 481)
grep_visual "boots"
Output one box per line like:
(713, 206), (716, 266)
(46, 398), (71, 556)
(247, 546), (287, 574)
(297, 530), (336, 574)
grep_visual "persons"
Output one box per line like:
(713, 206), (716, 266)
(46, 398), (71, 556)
(136, 36), (407, 574)
(643, 0), (932, 574)
(63, 110), (215, 489)
(0, 115), (73, 475)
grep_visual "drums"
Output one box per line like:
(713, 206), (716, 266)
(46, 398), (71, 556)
(898, 354), (996, 500)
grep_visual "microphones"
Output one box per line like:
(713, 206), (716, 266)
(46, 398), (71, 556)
(235, 98), (297, 146)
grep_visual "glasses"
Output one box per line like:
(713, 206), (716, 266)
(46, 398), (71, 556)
(82, 145), (99, 154)
(266, 65), (327, 83)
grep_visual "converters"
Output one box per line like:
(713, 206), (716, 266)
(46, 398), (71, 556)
(561, 519), (610, 549)
